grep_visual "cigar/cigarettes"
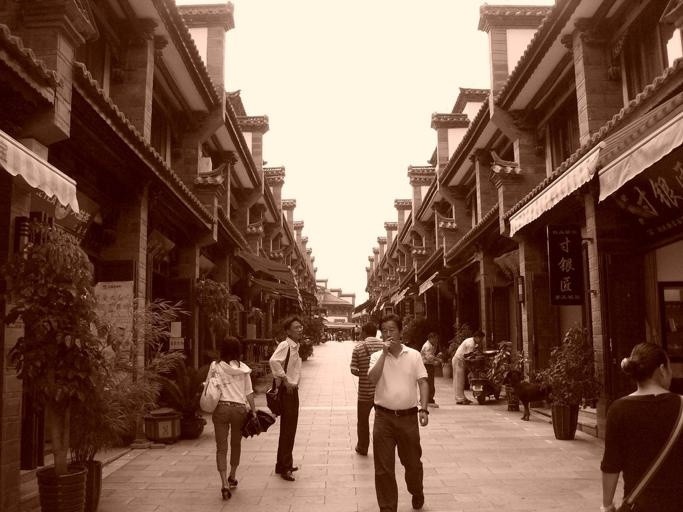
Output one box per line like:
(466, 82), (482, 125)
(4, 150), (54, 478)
(390, 339), (393, 342)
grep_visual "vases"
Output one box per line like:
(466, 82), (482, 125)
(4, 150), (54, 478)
(145, 411), (182, 444)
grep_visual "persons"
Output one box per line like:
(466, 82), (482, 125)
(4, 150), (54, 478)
(268, 317), (305, 482)
(600, 343), (683, 511)
(452, 332), (485, 404)
(206, 336), (257, 501)
(367, 312), (430, 511)
(351, 323), (384, 455)
(420, 332), (443, 409)
(322, 324), (360, 341)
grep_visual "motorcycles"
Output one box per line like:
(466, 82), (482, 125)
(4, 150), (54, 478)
(463, 350), (499, 404)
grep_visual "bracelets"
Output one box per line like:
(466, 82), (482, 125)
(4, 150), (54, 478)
(418, 407), (430, 414)
(600, 502), (615, 512)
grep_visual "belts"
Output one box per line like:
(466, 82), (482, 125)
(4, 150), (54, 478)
(373, 406), (418, 417)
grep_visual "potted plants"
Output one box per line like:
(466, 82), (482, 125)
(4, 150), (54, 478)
(71, 296), (190, 512)
(442, 343), (452, 379)
(535, 327), (599, 440)
(0, 225), (124, 512)
(486, 341), (533, 411)
(145, 355), (211, 438)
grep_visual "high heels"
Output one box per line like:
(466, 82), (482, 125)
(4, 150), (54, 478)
(221, 487), (231, 499)
(227, 476), (239, 489)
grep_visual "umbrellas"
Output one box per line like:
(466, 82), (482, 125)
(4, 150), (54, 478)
(241, 409), (275, 438)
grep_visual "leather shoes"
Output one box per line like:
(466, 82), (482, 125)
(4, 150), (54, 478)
(275, 467), (298, 481)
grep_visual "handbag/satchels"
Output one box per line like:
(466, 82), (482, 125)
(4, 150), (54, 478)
(266, 387), (282, 415)
(200, 375), (221, 413)
